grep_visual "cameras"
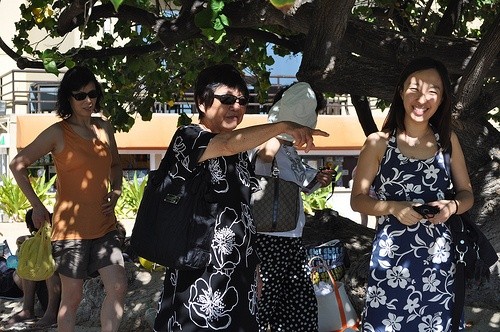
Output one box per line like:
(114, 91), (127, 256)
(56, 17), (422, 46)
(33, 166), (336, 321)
(413, 204), (440, 215)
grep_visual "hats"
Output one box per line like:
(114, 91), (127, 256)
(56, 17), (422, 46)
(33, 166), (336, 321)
(267, 82), (318, 143)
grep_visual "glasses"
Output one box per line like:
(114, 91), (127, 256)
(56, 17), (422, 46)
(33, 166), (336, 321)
(71, 90), (99, 100)
(214, 94), (247, 105)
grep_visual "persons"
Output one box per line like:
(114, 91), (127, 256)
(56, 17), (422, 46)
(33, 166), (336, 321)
(248, 83), (337, 332)
(9, 67), (128, 332)
(153, 63), (330, 332)
(8, 207), (126, 332)
(350, 60), (475, 332)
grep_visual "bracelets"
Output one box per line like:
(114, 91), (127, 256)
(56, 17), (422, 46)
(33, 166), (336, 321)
(454, 200), (459, 215)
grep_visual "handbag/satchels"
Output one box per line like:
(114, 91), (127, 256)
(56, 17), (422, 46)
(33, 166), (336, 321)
(306, 239), (346, 296)
(450, 215), (498, 289)
(17, 220), (58, 281)
(249, 150), (302, 232)
(129, 124), (218, 271)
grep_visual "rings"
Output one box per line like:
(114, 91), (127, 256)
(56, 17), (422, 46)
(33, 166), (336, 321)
(438, 221), (442, 225)
